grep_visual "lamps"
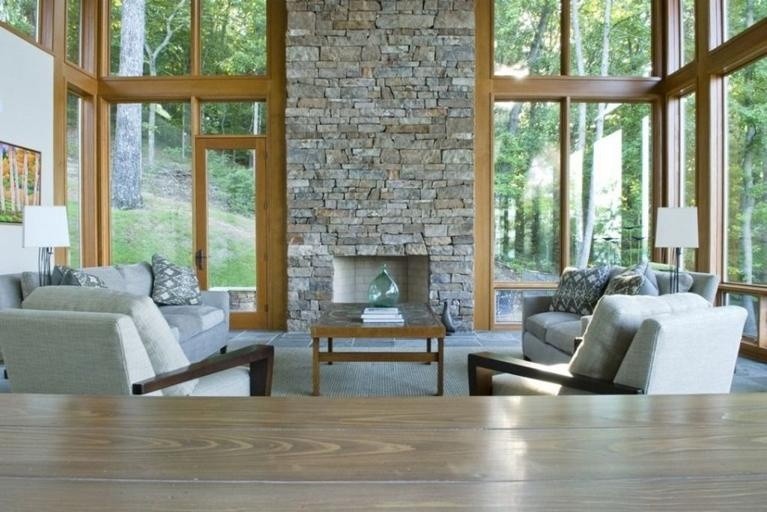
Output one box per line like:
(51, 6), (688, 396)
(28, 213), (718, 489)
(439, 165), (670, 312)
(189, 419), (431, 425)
(654, 207), (698, 293)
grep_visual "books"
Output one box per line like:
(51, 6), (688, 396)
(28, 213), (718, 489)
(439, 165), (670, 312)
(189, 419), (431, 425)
(361, 307), (404, 322)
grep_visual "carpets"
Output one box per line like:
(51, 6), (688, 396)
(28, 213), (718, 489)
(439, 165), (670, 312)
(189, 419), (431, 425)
(206, 347), (524, 396)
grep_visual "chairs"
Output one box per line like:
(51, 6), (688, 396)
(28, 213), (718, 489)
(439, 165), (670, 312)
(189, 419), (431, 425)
(1, 286), (273, 396)
(467, 295), (749, 395)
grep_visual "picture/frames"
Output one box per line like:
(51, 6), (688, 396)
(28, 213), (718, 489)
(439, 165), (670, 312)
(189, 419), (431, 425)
(0, 141), (41, 224)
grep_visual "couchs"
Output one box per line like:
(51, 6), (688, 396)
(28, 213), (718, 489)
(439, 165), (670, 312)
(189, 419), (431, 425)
(1, 260), (229, 364)
(523, 262), (722, 366)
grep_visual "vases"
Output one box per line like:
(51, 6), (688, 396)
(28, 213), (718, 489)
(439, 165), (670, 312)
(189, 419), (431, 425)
(23, 205), (71, 286)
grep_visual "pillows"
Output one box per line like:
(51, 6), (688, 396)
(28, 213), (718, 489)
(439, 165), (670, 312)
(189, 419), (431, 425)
(22, 252), (203, 396)
(549, 253), (694, 381)
